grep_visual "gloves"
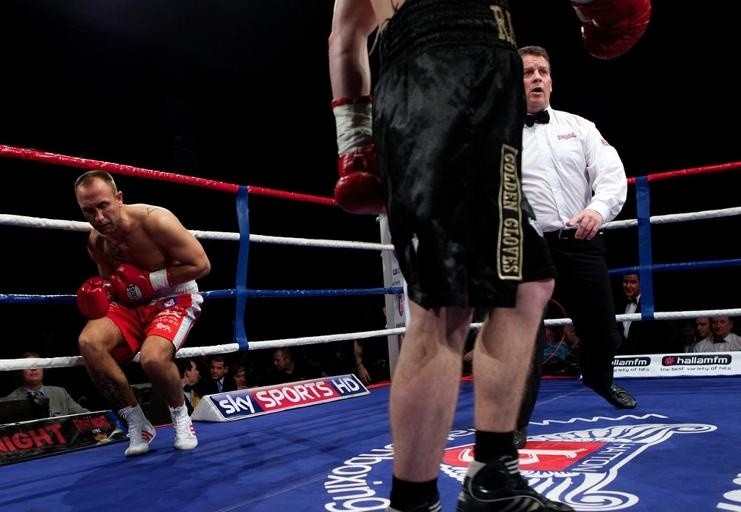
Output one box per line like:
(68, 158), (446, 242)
(331, 95), (388, 217)
(77, 277), (111, 318)
(572, 1), (652, 61)
(110, 265), (174, 306)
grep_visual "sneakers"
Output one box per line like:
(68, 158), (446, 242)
(457, 453), (573, 512)
(169, 403), (198, 450)
(125, 406), (157, 458)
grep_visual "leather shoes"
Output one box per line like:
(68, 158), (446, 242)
(582, 378), (637, 409)
(513, 426), (527, 449)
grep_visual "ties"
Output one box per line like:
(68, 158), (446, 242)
(35, 389), (53, 417)
(185, 386), (201, 410)
(217, 379), (223, 393)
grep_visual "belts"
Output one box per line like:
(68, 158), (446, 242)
(543, 228), (577, 242)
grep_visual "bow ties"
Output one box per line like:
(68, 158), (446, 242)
(523, 110), (551, 127)
(713, 338), (725, 343)
(626, 297), (638, 305)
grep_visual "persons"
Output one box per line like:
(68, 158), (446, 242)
(464, 273), (740, 376)
(512, 46), (637, 449)
(75, 171), (212, 457)
(328, 0), (650, 512)
(0, 304), (392, 419)
